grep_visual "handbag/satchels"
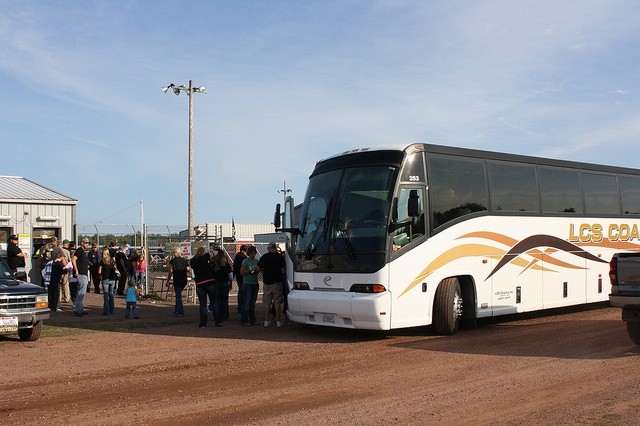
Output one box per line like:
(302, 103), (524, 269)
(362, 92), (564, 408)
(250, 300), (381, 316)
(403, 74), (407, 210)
(208, 251), (224, 276)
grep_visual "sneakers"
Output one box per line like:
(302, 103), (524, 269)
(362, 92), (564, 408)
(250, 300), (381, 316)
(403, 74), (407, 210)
(265, 321), (272, 328)
(277, 320), (284, 328)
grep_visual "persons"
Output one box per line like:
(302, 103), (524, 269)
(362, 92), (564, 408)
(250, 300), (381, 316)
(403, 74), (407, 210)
(258, 240), (288, 328)
(240, 246), (260, 326)
(234, 244), (248, 314)
(211, 249), (233, 321)
(6, 234), (29, 280)
(35, 235), (145, 319)
(166, 247), (190, 318)
(190, 247), (224, 327)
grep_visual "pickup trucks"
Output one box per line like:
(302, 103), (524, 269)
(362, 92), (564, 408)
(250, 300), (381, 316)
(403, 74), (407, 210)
(608, 253), (640, 344)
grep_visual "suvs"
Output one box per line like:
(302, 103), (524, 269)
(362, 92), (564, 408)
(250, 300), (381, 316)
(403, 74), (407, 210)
(0, 259), (48, 341)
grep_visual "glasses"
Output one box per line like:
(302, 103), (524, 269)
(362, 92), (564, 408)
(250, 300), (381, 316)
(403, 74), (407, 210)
(84, 242), (90, 245)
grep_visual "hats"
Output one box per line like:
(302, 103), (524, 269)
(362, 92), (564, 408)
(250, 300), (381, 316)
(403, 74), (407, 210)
(63, 239), (71, 244)
(81, 237), (90, 241)
(9, 235), (19, 240)
(50, 237), (57, 241)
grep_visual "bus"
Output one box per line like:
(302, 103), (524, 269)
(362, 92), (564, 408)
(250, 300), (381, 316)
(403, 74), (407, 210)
(275, 142), (640, 335)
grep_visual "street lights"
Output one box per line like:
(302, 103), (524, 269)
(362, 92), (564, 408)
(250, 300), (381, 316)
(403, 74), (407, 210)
(161, 84), (206, 237)
